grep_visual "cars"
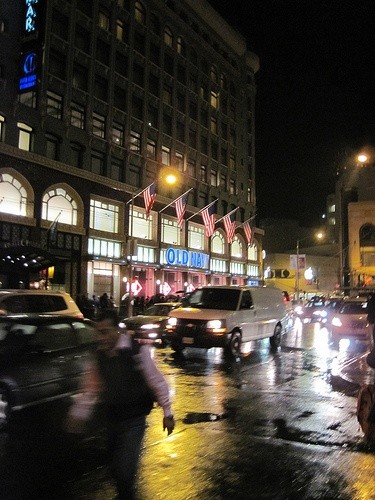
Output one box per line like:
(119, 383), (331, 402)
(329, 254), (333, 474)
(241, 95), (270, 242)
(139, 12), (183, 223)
(0, 315), (118, 430)
(118, 301), (181, 347)
(299, 294), (375, 341)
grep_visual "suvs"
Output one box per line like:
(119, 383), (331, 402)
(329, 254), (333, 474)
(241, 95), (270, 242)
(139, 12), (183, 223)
(0, 289), (85, 320)
(165, 285), (290, 362)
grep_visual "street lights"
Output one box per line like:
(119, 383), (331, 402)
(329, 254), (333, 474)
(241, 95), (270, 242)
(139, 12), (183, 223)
(127, 172), (181, 315)
(296, 233), (323, 293)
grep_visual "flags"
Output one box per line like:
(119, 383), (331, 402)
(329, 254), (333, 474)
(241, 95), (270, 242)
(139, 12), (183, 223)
(143, 183), (157, 221)
(222, 210), (237, 243)
(174, 192), (189, 228)
(243, 216), (256, 243)
(200, 201), (218, 239)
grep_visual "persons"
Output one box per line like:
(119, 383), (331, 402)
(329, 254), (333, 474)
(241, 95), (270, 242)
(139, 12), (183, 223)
(75, 292), (179, 326)
(66, 309), (175, 500)
(311, 294), (325, 306)
